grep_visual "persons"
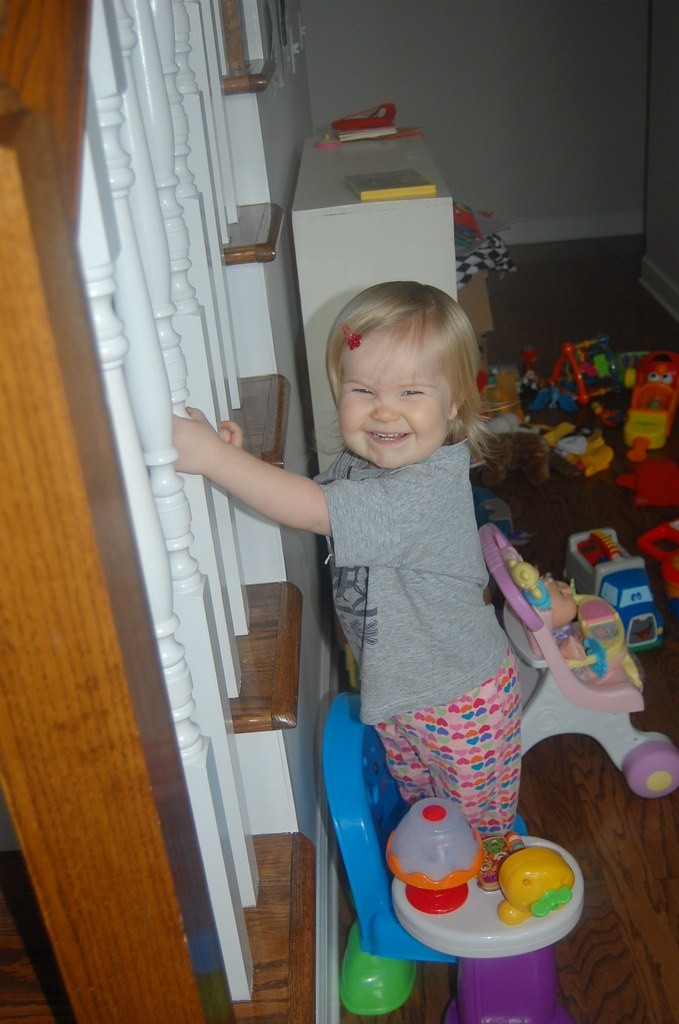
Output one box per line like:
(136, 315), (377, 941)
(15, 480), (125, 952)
(169, 281), (526, 892)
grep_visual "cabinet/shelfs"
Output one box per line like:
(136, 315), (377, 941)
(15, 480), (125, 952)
(290, 122), (460, 475)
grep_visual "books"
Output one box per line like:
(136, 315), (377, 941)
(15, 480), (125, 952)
(344, 168), (436, 200)
(330, 122), (398, 142)
